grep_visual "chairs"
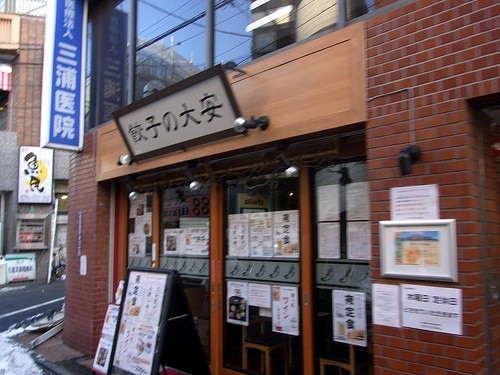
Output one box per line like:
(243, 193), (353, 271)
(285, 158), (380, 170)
(319, 344), (370, 375)
(242, 317), (292, 375)
